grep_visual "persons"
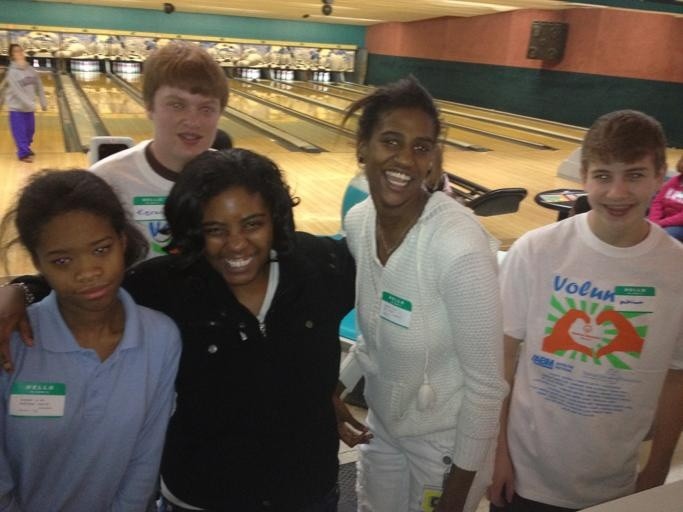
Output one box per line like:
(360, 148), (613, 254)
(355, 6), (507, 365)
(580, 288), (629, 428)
(331, 79), (505, 512)
(0, 146), (357, 512)
(649, 155), (683, 239)
(86, 40), (231, 272)
(0, 166), (184, 511)
(485, 110), (682, 512)
(420, 145), (453, 198)
(4, 42), (47, 163)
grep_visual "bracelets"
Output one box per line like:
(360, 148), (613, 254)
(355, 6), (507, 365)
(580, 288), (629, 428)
(5, 281), (34, 303)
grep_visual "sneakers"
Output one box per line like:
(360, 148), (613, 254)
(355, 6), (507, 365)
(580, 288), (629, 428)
(18, 155), (34, 164)
